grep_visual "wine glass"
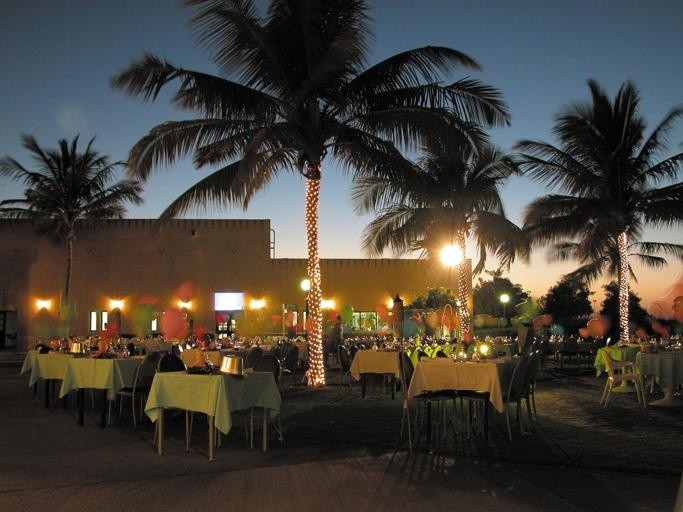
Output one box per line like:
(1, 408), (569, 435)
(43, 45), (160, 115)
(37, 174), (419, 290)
(202, 335), (264, 349)
(354, 335), (439, 351)
(635, 331), (683, 352)
(482, 334), (583, 344)
(446, 343), (481, 364)
(57, 335), (166, 357)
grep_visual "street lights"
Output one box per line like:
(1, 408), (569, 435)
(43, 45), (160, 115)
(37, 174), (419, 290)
(499, 293), (509, 315)
(301, 278), (310, 347)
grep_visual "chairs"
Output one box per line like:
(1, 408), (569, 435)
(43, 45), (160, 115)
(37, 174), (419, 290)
(397, 334), (540, 462)
(594, 334), (682, 409)
(145, 334), (308, 463)
(322, 333), (413, 400)
(19, 334), (155, 428)
(538, 334), (605, 368)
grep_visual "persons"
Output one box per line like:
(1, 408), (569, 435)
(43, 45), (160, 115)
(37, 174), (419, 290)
(332, 316), (343, 351)
(442, 305), (457, 336)
